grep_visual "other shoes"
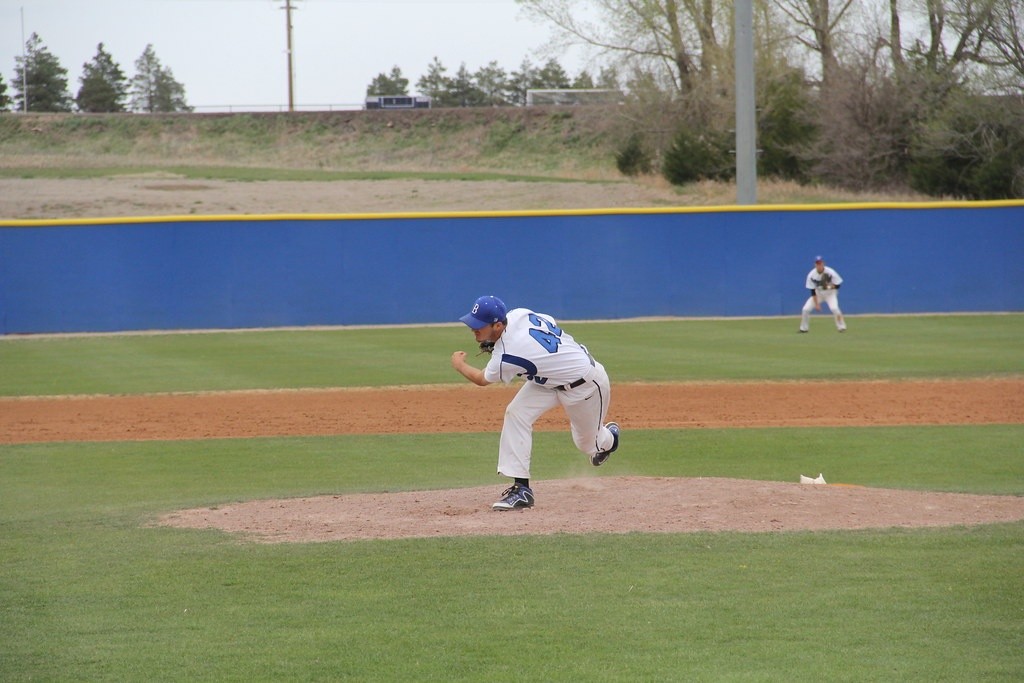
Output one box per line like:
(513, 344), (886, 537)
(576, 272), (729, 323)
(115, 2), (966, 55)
(795, 329), (807, 333)
(840, 329), (847, 334)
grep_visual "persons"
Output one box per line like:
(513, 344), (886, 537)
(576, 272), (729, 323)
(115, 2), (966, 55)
(451, 295), (620, 511)
(798, 256), (847, 333)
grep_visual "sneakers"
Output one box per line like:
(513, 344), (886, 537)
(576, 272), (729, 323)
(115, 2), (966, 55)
(589, 422), (620, 466)
(493, 483), (535, 511)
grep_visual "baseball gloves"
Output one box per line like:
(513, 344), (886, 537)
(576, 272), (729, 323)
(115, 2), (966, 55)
(821, 273), (828, 289)
(473, 339), (496, 356)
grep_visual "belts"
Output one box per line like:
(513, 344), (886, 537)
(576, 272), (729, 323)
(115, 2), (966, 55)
(558, 354), (596, 391)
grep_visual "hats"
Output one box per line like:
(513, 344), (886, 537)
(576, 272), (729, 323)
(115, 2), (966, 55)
(814, 255), (824, 263)
(459, 295), (507, 329)
